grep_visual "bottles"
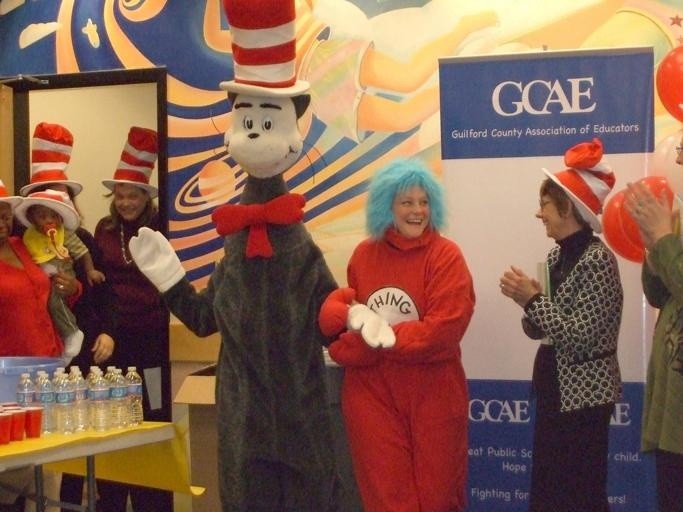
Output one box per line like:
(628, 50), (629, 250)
(16, 373), (34, 408)
(87, 366), (100, 383)
(108, 368), (127, 430)
(125, 366), (143, 427)
(68, 366), (81, 384)
(53, 367), (65, 377)
(33, 371), (47, 384)
(87, 371), (110, 434)
(105, 367), (119, 381)
(33, 374), (54, 432)
(69, 371), (90, 432)
(53, 374), (76, 435)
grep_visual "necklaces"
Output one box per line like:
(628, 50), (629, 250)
(116, 217), (135, 268)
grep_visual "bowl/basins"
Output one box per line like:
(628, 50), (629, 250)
(0, 356), (68, 403)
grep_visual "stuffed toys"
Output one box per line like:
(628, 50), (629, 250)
(129, 0), (396, 512)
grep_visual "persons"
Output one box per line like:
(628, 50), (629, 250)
(621, 122), (683, 512)
(21, 193), (105, 367)
(96, 127), (182, 512)
(498, 141), (627, 512)
(18, 111), (98, 295)
(0, 178), (81, 365)
(328, 157), (476, 512)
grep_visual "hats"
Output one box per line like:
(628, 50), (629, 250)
(1, 182), (23, 208)
(542, 139), (616, 232)
(220, 0), (309, 96)
(21, 123), (83, 195)
(17, 189), (79, 231)
(104, 127), (158, 196)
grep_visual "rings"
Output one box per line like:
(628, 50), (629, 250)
(59, 284), (64, 291)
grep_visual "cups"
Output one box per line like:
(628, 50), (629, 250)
(18, 406), (44, 438)
(2, 409), (24, 441)
(0, 413), (13, 445)
(0, 401), (22, 412)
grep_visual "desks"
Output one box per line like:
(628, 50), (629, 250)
(0, 421), (177, 512)
